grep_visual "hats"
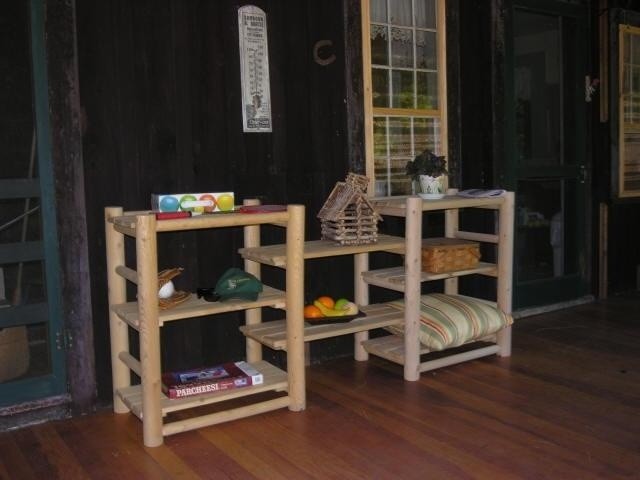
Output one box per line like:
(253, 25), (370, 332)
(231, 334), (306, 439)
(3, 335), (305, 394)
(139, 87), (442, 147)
(215, 267), (263, 303)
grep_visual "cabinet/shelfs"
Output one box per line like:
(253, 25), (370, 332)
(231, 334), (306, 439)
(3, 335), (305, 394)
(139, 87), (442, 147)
(516, 177), (585, 309)
(105, 189), (515, 447)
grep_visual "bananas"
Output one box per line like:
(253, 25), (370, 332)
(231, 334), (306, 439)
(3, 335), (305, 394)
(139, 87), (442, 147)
(313, 301), (351, 316)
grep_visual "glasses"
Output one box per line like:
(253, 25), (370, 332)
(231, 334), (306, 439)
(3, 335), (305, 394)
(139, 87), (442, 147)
(197, 287), (219, 302)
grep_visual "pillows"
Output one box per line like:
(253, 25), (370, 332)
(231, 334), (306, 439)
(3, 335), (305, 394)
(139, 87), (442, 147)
(382, 293), (515, 352)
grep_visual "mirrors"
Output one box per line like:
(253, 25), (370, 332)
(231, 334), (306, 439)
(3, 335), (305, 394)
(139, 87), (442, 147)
(512, 5), (563, 167)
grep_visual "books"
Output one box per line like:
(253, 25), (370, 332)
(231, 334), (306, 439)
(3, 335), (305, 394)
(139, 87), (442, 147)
(160, 360), (264, 401)
(457, 187), (506, 198)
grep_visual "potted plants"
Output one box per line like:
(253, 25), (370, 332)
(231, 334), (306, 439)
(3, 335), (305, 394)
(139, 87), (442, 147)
(405, 148), (450, 200)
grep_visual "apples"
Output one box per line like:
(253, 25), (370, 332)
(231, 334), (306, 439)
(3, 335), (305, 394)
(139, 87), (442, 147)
(345, 302), (358, 316)
(335, 298), (350, 309)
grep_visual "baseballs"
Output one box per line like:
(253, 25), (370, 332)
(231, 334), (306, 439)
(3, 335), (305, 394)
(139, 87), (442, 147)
(159, 281), (175, 299)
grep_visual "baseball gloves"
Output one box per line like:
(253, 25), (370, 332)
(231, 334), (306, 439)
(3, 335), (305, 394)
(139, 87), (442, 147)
(136, 267), (192, 308)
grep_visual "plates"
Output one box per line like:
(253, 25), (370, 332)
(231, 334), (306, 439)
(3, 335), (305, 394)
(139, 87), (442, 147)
(305, 310), (367, 324)
(418, 193), (446, 200)
